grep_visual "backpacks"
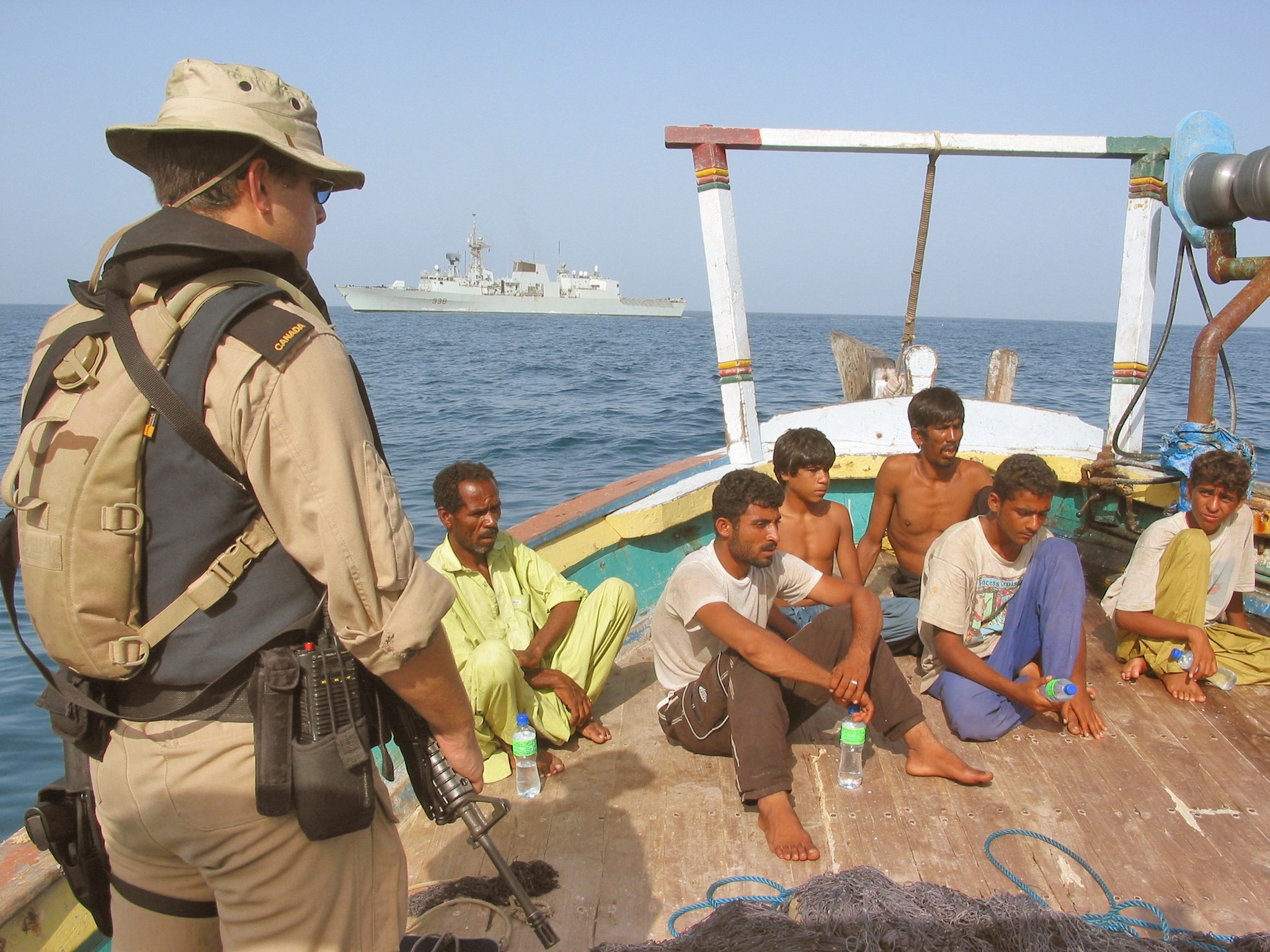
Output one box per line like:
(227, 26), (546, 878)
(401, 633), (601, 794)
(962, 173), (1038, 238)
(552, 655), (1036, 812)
(0, 267), (328, 682)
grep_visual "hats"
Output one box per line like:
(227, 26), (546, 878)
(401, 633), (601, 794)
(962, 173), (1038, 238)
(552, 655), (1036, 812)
(105, 60), (366, 193)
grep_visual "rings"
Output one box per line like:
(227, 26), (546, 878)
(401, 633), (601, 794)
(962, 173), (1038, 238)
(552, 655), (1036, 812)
(850, 680), (858, 685)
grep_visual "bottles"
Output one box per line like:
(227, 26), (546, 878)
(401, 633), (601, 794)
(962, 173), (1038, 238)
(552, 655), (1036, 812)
(1038, 679), (1078, 703)
(513, 713), (540, 798)
(1171, 649), (1238, 690)
(837, 701), (866, 790)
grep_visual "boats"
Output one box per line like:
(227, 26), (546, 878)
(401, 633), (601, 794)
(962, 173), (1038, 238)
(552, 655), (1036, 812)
(334, 213), (687, 318)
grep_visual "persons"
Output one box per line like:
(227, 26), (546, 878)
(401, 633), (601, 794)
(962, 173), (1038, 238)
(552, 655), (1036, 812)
(646, 467), (993, 860)
(424, 464), (633, 778)
(1099, 449), (1270, 701)
(0, 63), (483, 952)
(765, 427), (919, 656)
(854, 387), (995, 598)
(919, 451), (1108, 741)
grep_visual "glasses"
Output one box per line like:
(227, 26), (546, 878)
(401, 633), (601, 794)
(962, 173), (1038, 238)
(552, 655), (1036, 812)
(278, 163), (334, 204)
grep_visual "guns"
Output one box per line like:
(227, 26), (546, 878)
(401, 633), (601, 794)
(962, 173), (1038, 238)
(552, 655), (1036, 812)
(23, 794), (114, 938)
(351, 661), (566, 949)
